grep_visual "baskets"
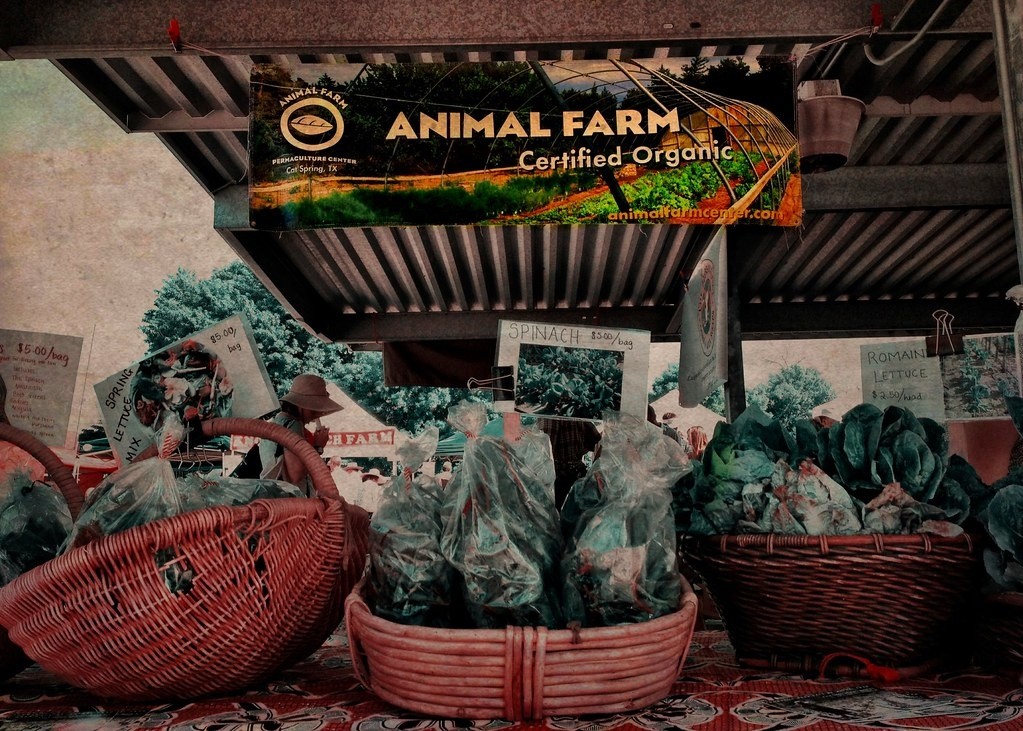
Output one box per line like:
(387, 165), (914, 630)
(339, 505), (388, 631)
(346, 570), (699, 722)
(0, 424), (104, 682)
(683, 535), (975, 679)
(0, 419), (370, 701)
(967, 591), (1023, 677)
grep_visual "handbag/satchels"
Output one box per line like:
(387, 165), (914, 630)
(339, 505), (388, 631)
(228, 444), (263, 479)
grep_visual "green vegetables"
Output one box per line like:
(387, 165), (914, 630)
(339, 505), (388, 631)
(0, 465), (308, 598)
(678, 396), (1023, 596)
(366, 435), (682, 630)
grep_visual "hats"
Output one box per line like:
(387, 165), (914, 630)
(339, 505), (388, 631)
(279, 375), (344, 416)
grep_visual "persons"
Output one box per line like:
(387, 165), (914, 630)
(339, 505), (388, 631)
(259, 375), (345, 498)
(323, 456), (454, 520)
(814, 409), (842, 429)
(648, 403), (709, 463)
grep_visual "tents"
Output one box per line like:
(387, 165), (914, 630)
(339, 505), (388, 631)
(232, 383), (529, 506)
(596, 389), (726, 442)
(812, 388), (863, 427)
(44, 420), (229, 497)
